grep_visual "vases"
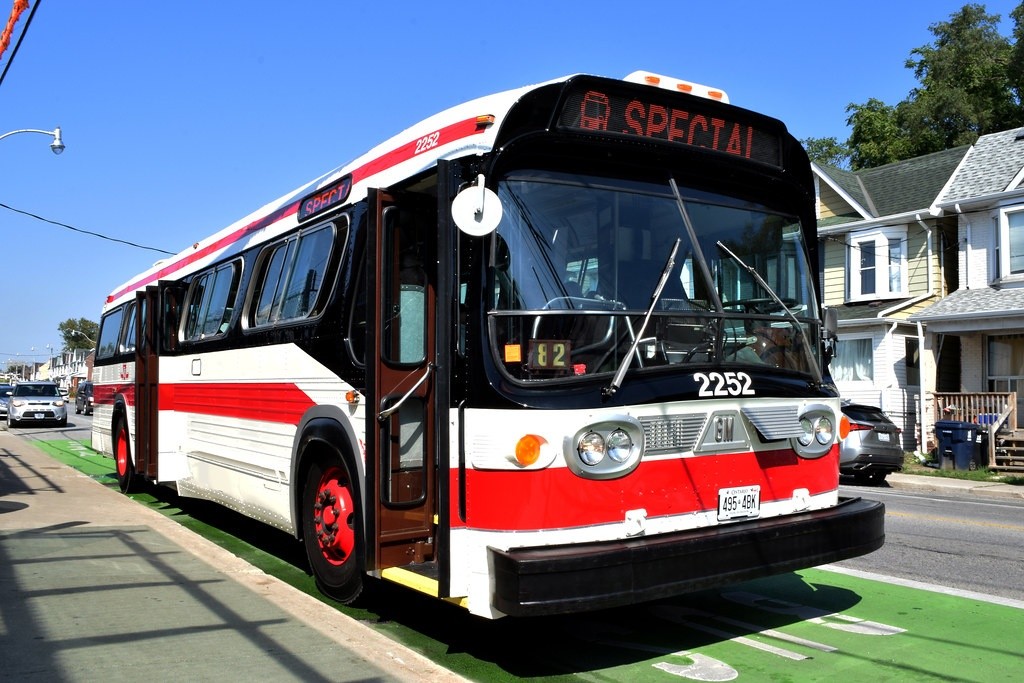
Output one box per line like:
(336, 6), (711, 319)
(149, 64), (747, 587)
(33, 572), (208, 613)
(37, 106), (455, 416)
(944, 413), (962, 421)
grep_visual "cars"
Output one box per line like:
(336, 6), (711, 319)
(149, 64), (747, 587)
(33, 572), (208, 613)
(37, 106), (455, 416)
(5, 382), (69, 428)
(0, 382), (70, 417)
(74, 381), (94, 415)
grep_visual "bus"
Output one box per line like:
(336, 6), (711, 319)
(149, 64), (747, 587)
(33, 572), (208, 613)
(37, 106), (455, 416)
(90, 71), (888, 622)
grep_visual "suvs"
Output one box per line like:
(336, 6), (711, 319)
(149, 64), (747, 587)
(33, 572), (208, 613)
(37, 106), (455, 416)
(840, 395), (904, 483)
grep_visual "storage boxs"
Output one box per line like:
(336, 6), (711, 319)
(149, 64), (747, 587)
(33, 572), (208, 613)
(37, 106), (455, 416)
(979, 413), (1002, 424)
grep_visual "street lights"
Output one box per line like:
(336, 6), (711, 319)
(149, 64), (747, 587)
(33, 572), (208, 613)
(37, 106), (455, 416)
(16, 352), (25, 381)
(45, 344), (53, 383)
(8, 357), (19, 383)
(30, 346), (36, 382)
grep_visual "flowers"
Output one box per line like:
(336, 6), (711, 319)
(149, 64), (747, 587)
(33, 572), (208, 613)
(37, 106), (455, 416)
(941, 404), (961, 414)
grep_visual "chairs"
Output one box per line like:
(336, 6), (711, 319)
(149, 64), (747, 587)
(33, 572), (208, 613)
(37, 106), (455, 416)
(20, 387), (31, 394)
(42, 386), (53, 394)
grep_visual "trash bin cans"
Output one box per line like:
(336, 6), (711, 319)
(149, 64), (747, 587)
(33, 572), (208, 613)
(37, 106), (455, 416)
(934, 420), (983, 473)
